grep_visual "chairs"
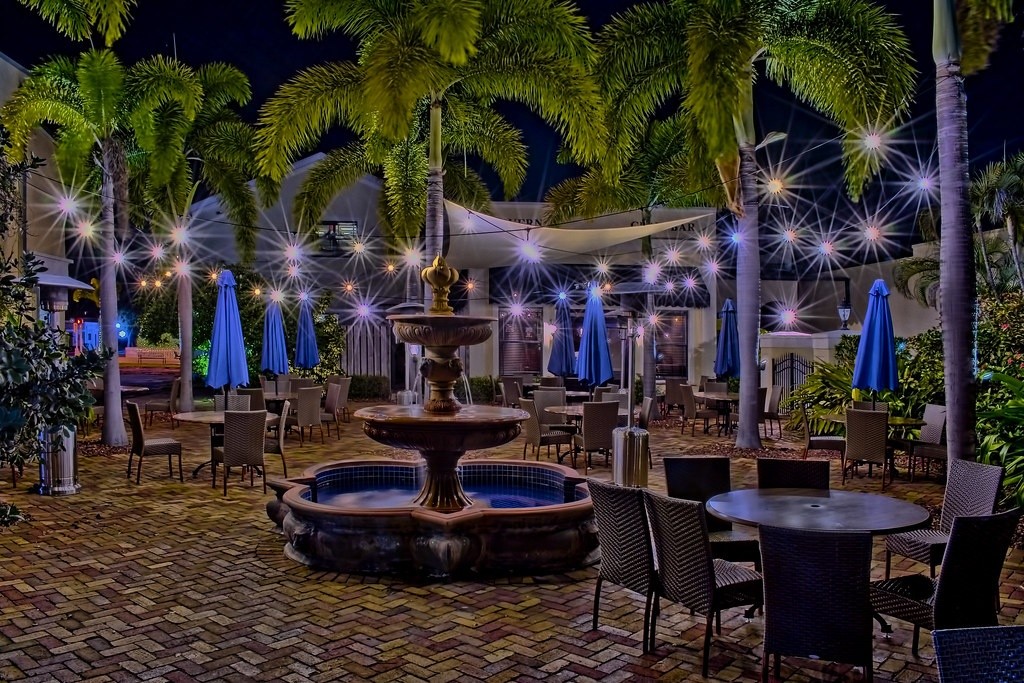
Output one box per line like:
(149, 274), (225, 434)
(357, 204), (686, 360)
(173, 352), (180, 361)
(497, 375), (1024, 683)
(82, 374), (352, 498)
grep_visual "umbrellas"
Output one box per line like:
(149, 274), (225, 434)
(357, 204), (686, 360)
(205, 270), (319, 411)
(851, 279), (899, 410)
(547, 281), (613, 402)
(713, 298), (740, 397)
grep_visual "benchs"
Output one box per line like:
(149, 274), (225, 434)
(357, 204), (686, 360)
(138, 352), (166, 367)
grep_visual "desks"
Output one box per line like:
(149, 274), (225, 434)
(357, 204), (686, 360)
(88, 385), (151, 429)
(693, 392), (741, 440)
(171, 411), (280, 479)
(262, 392), (325, 442)
(819, 414), (928, 479)
(542, 406), (638, 470)
(706, 487), (931, 683)
(565, 391), (594, 425)
